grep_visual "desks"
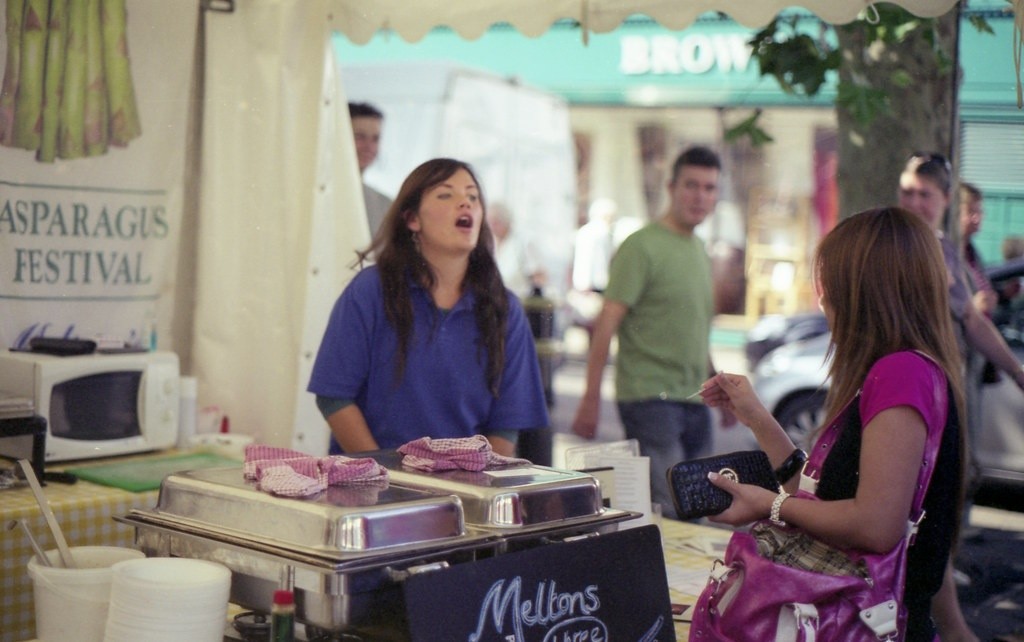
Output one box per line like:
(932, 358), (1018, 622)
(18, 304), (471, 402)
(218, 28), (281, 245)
(222, 506), (731, 642)
(1, 432), (273, 642)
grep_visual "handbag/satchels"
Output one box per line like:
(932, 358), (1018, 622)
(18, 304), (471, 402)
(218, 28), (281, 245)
(399, 524), (678, 642)
(688, 493), (917, 642)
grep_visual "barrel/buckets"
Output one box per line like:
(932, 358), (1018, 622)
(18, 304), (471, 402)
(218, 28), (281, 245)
(27, 545), (146, 642)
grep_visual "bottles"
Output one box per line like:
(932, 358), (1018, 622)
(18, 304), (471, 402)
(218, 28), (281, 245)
(270, 589), (298, 642)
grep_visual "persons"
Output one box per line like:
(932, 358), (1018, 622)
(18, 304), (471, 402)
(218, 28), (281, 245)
(348, 103), (392, 265)
(959, 183), (1020, 320)
(306, 158), (550, 625)
(570, 146), (739, 528)
(697, 206), (969, 642)
(896, 151), (1023, 390)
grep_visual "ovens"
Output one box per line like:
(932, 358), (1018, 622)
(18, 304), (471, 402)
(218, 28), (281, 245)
(1, 341), (181, 463)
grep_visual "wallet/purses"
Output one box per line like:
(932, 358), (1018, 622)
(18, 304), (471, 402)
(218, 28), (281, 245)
(666, 450), (780, 518)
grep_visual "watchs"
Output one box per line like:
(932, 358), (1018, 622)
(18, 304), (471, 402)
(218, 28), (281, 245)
(767, 492), (791, 528)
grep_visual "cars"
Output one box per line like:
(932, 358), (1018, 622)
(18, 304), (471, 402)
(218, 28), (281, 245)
(746, 254), (1024, 486)
(745, 313), (831, 372)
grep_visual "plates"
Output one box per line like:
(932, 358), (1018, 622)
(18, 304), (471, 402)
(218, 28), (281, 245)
(103, 557), (232, 642)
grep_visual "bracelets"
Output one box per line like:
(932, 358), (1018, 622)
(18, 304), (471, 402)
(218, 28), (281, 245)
(770, 448), (808, 485)
